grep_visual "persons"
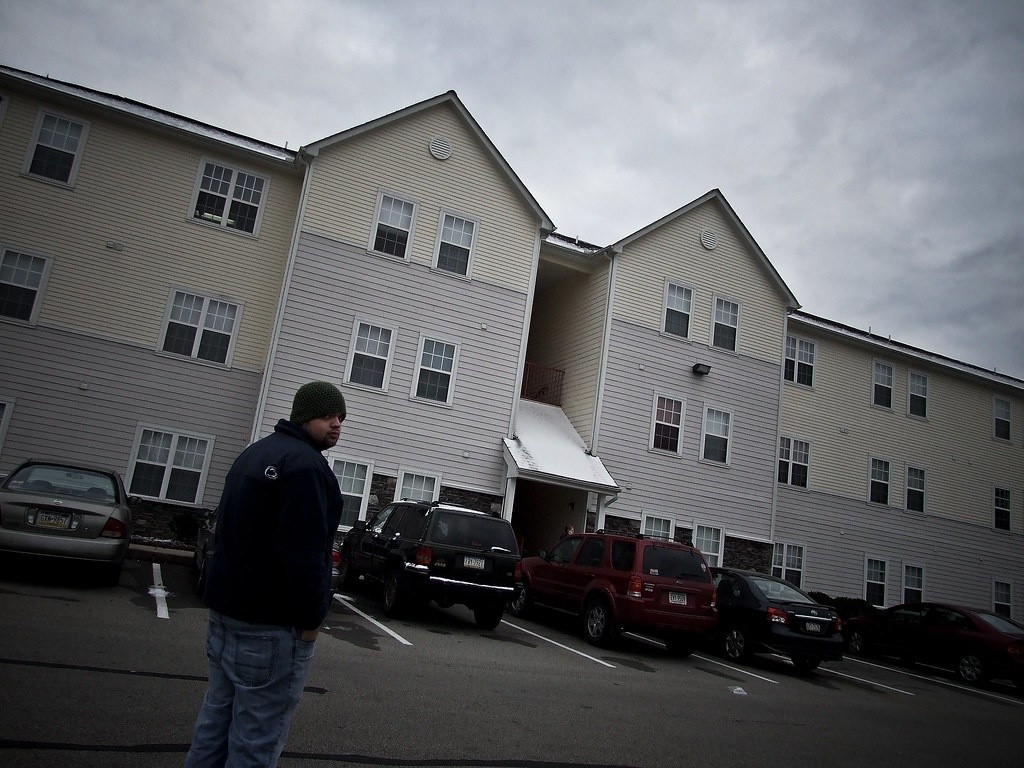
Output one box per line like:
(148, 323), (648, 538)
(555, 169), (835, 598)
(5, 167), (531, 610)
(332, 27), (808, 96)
(183, 382), (349, 768)
(559, 524), (575, 542)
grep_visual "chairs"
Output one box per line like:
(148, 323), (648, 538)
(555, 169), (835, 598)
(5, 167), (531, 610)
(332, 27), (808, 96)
(86, 486), (108, 501)
(579, 542), (603, 561)
(718, 580), (731, 593)
(34, 480), (52, 493)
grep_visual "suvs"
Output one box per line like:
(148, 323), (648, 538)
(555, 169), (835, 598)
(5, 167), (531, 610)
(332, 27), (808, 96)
(343, 496), (525, 630)
(507, 529), (717, 658)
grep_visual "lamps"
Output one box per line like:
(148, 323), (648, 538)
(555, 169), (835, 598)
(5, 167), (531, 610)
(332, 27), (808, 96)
(693, 363), (711, 375)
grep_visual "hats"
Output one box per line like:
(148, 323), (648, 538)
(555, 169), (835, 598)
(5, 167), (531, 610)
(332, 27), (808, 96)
(291, 381), (346, 424)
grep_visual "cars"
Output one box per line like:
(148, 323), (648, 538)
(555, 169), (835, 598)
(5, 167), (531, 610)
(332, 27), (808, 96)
(842, 601), (1024, 687)
(193, 503), (346, 597)
(0, 456), (145, 585)
(707, 566), (843, 669)
